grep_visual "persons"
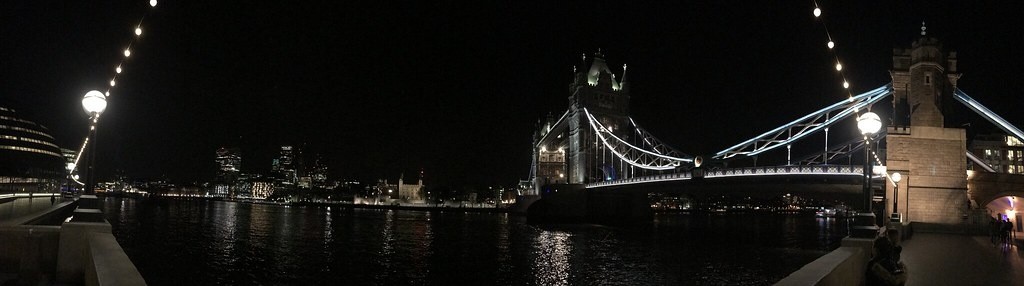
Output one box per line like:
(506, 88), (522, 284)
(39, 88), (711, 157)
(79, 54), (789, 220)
(50, 193), (56, 206)
(28, 191), (33, 201)
(870, 229), (902, 286)
(990, 214), (1013, 246)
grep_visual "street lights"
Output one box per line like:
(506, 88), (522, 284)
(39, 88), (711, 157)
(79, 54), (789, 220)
(890, 173), (902, 222)
(66, 162), (79, 197)
(72, 90), (108, 221)
(847, 110), (881, 236)
